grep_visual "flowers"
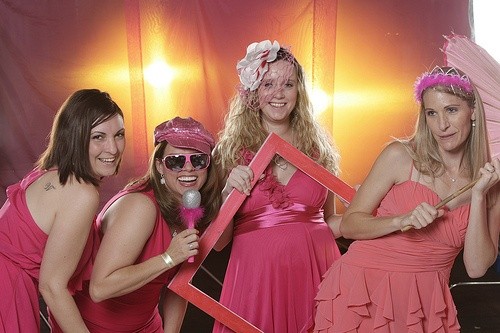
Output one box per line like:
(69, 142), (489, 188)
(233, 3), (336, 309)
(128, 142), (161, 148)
(236, 40), (280, 92)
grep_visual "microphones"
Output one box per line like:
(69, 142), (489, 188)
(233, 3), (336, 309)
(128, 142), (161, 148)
(183, 189), (201, 262)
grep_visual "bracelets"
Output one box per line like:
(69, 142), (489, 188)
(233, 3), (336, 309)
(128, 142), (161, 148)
(161, 252), (176, 268)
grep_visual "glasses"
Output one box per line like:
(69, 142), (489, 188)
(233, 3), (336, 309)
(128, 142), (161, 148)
(160, 153), (211, 173)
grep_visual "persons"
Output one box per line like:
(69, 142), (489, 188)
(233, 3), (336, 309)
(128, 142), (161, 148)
(0, 88), (127, 332)
(205, 38), (364, 332)
(46, 115), (215, 333)
(314, 64), (499, 333)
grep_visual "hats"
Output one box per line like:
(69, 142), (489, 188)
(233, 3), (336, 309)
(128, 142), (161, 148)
(154, 114), (215, 155)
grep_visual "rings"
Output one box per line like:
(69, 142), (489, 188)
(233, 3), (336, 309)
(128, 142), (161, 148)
(488, 169), (495, 173)
(189, 243), (193, 249)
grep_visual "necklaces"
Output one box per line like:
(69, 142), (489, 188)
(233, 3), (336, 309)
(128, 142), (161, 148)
(440, 160), (465, 183)
(272, 154), (290, 171)
(169, 223), (178, 237)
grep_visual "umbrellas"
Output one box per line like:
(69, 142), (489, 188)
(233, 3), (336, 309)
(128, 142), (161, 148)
(401, 30), (499, 233)
